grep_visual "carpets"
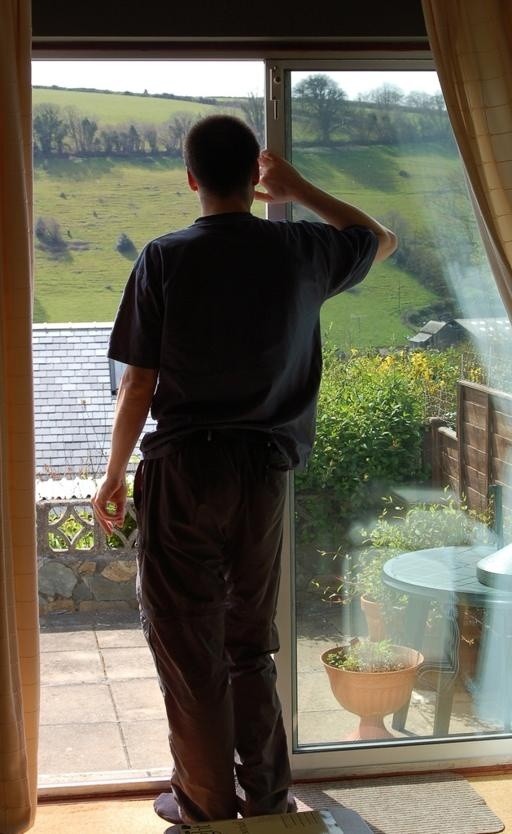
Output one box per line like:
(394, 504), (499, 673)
(174, 772), (506, 834)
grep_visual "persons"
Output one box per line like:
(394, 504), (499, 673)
(92, 113), (401, 831)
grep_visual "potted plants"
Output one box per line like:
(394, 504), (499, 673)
(322, 644), (424, 741)
(355, 523), (425, 644)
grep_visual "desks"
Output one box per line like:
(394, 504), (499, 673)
(384, 546), (512, 737)
